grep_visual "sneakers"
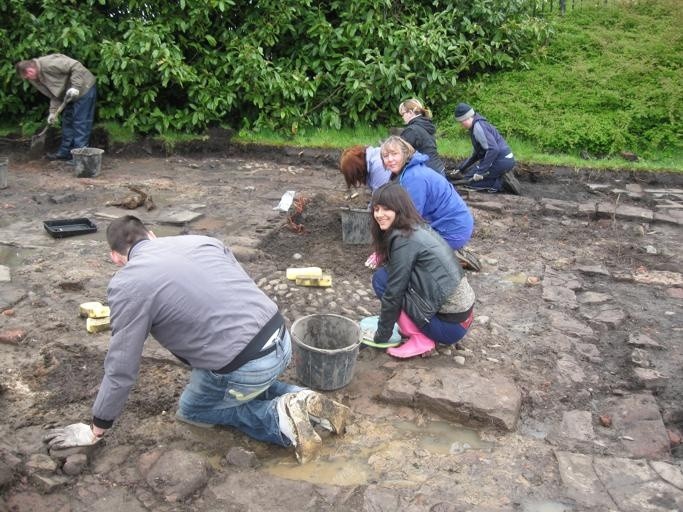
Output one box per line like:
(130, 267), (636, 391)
(505, 171), (522, 195)
(453, 246), (482, 272)
(275, 392), (321, 464)
(301, 390), (348, 435)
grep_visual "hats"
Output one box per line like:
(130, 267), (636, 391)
(454, 102), (475, 121)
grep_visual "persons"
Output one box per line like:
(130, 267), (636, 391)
(339, 144), (393, 214)
(447, 103), (522, 195)
(398, 98), (448, 178)
(41, 215), (356, 466)
(361, 182), (476, 360)
(16, 53), (98, 166)
(363, 135), (481, 272)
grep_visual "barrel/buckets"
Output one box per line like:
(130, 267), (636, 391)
(70, 147), (104, 178)
(340, 206), (375, 246)
(0, 157), (8, 190)
(290, 313), (363, 391)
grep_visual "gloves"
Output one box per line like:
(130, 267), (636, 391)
(472, 173), (483, 181)
(66, 87), (79, 98)
(43, 422), (102, 449)
(48, 114), (56, 124)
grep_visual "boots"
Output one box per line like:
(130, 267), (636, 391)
(387, 310), (437, 359)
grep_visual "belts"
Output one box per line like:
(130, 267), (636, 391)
(251, 322), (286, 359)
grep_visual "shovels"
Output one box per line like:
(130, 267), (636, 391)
(29, 95), (73, 151)
